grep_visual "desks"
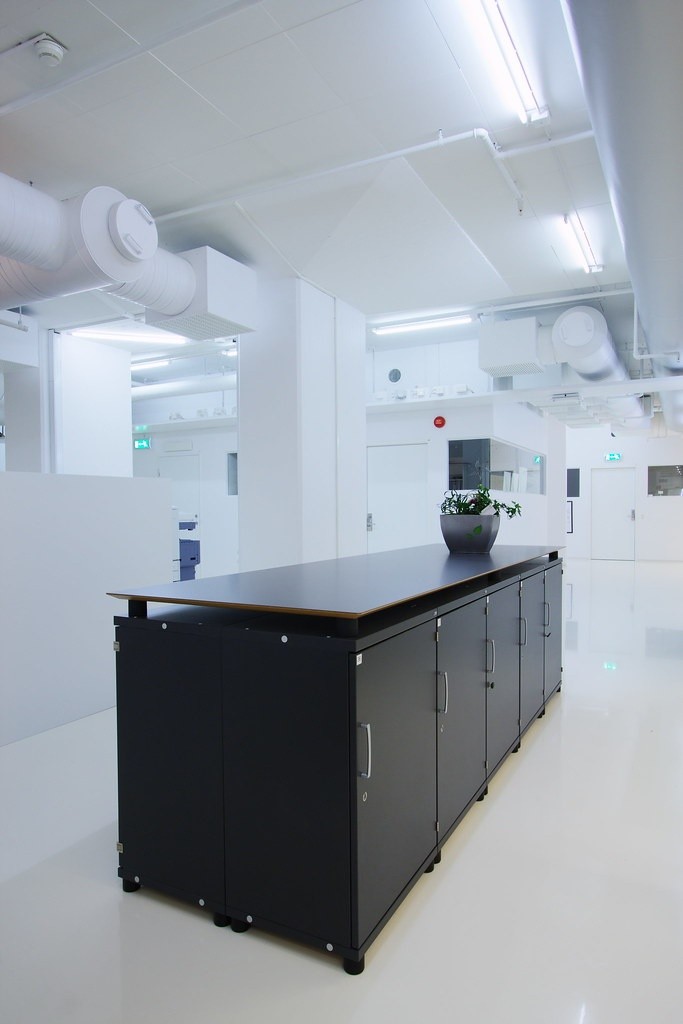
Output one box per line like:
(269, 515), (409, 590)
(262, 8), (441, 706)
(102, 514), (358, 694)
(107, 543), (566, 975)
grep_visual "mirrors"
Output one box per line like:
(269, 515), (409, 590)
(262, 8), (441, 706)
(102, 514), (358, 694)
(647, 464), (683, 496)
(448, 439), (545, 492)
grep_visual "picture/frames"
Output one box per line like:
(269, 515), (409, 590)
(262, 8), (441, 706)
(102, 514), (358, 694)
(566, 501), (573, 533)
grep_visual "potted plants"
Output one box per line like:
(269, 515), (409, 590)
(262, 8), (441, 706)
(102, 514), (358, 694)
(439, 484), (522, 554)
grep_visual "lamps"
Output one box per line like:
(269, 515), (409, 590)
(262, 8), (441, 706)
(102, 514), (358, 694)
(481, 0), (549, 125)
(562, 214), (603, 274)
